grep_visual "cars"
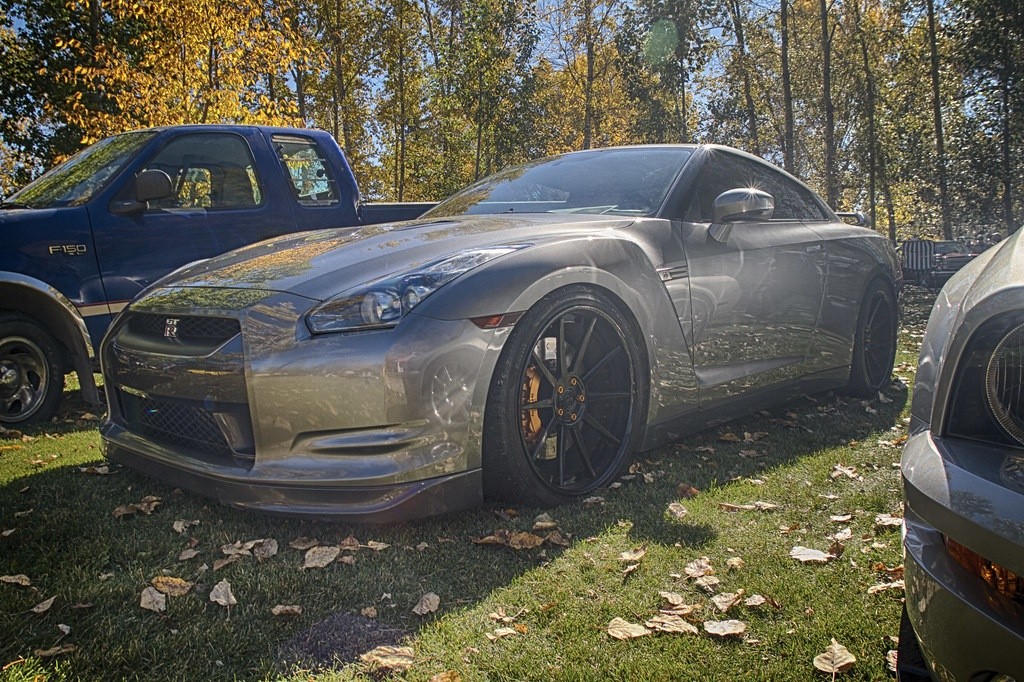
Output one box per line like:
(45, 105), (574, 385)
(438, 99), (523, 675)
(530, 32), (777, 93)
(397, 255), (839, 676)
(923, 240), (980, 288)
(98, 143), (904, 525)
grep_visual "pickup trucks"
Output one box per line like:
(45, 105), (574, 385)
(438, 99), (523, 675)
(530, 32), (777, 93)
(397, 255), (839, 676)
(0, 125), (567, 432)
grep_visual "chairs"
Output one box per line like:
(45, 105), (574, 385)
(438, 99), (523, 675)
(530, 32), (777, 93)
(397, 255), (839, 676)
(899, 240), (940, 294)
(148, 166), (179, 209)
(211, 161), (257, 208)
